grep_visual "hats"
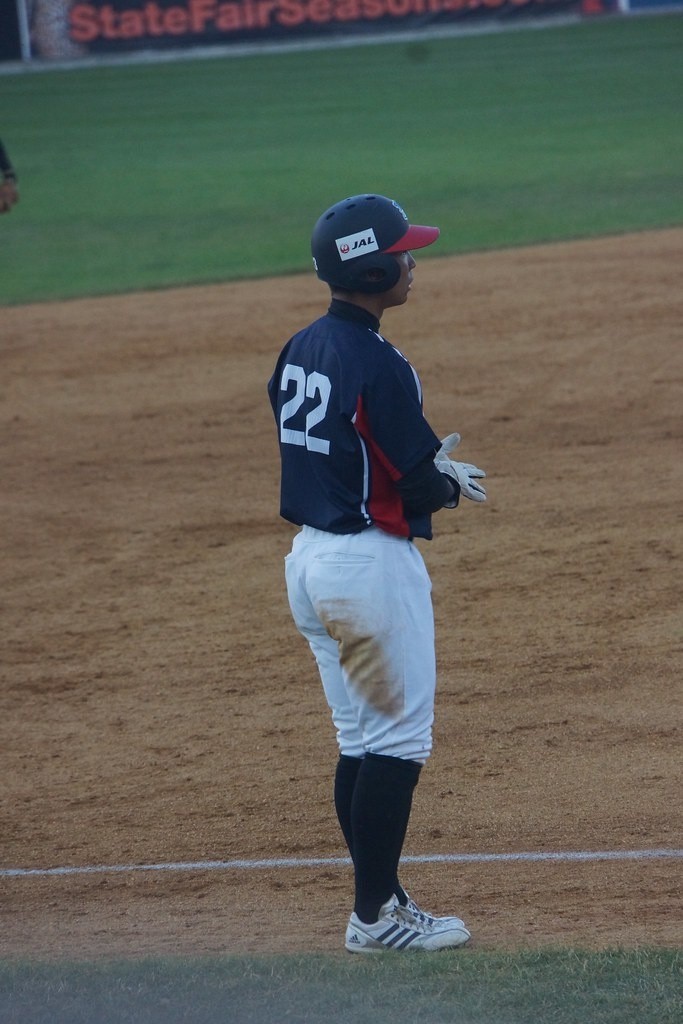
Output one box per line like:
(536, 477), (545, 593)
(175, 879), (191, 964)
(311, 194), (440, 281)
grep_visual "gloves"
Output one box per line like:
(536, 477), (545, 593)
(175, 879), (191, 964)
(432, 431), (488, 510)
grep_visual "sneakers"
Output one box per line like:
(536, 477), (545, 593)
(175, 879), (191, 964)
(345, 893), (470, 948)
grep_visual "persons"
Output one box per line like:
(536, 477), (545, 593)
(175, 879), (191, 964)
(0, 143), (19, 214)
(268, 196), (486, 955)
